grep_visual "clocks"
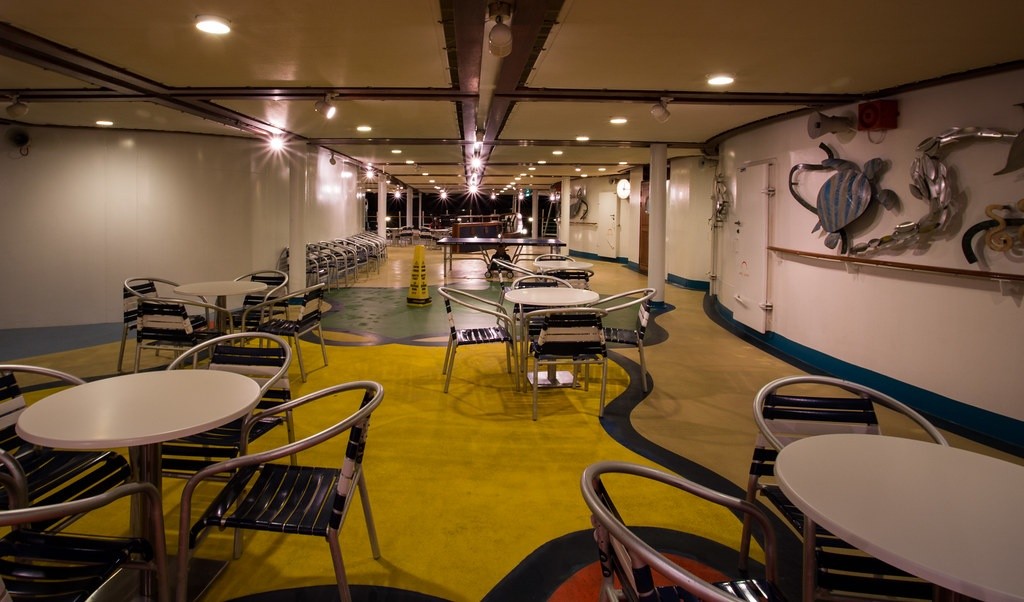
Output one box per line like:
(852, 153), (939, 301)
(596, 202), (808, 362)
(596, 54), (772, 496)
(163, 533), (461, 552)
(616, 179), (630, 199)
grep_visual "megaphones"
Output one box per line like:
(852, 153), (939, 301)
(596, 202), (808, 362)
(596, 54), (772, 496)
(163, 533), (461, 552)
(807, 111), (852, 139)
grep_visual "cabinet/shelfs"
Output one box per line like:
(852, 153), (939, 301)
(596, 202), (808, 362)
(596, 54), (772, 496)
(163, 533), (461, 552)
(450, 222), (500, 254)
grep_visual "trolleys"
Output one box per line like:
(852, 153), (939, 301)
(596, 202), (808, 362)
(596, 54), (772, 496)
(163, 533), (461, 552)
(478, 238), (514, 279)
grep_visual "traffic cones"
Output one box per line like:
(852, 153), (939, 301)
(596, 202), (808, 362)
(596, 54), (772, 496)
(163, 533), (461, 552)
(404, 245), (432, 307)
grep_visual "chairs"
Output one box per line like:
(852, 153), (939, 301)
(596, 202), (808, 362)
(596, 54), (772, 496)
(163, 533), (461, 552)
(0, 213), (1024, 602)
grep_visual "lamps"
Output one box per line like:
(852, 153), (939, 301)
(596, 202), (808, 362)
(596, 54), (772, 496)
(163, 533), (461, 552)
(489, 0), (512, 57)
(705, 72), (736, 86)
(386, 176), (391, 184)
(194, 14), (231, 33)
(330, 152), (336, 165)
(313, 93), (336, 119)
(650, 97), (673, 123)
(6, 96), (28, 119)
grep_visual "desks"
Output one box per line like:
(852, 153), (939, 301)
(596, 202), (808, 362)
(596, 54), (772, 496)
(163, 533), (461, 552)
(774, 433), (1024, 602)
(434, 229), (449, 249)
(174, 281), (268, 347)
(505, 287), (600, 387)
(390, 228), (403, 246)
(16, 370), (262, 602)
(533, 261), (593, 270)
(437, 237), (567, 276)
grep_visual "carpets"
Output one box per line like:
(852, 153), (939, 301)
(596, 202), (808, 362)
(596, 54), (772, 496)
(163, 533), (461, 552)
(0, 287), (818, 602)
